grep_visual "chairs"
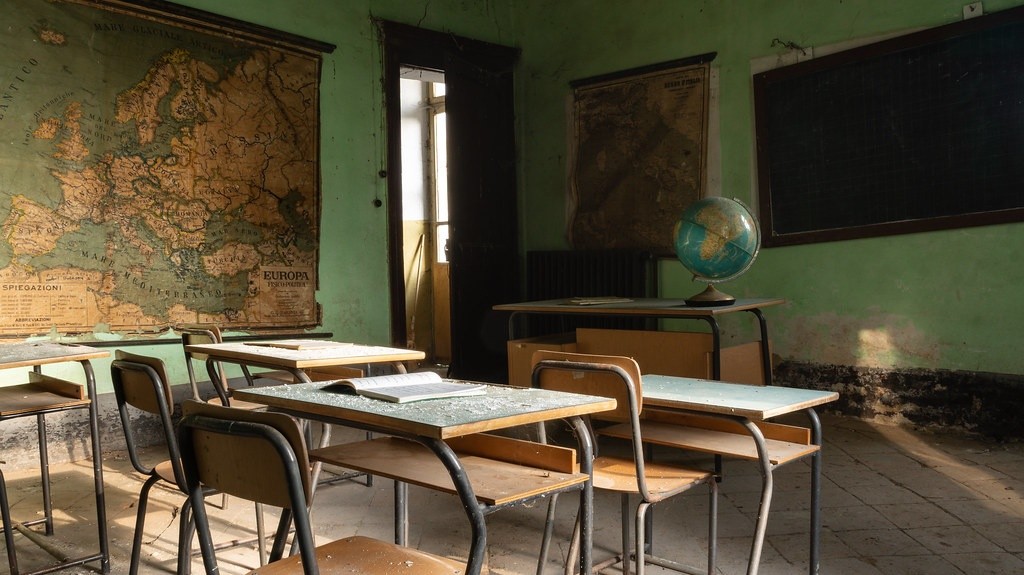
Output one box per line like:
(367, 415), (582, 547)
(181, 322), (254, 402)
(530, 349), (720, 575)
(110, 350), (267, 575)
(175, 400), (488, 575)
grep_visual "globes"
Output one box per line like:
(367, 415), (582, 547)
(672, 195), (763, 306)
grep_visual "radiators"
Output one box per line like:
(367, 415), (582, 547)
(525, 250), (658, 337)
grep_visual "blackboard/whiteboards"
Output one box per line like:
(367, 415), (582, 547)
(747, 3), (1024, 249)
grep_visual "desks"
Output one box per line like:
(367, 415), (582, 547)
(233, 378), (617, 575)
(0, 339), (112, 575)
(492, 296), (787, 484)
(564, 373), (839, 575)
(186, 339), (425, 546)
(222, 333), (334, 386)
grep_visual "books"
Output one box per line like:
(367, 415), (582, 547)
(565, 297), (634, 306)
(243, 338), (354, 351)
(317, 371), (488, 404)
